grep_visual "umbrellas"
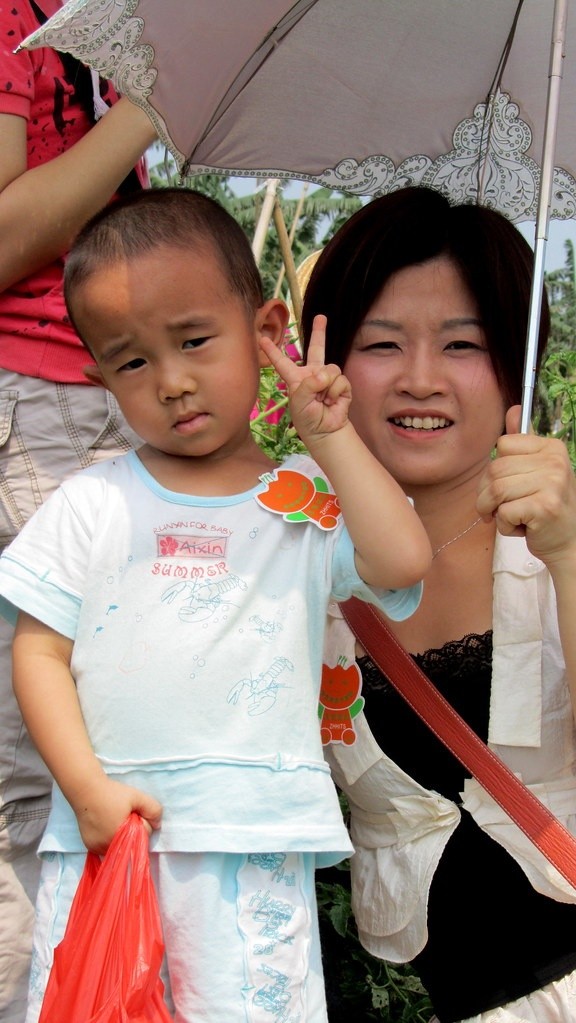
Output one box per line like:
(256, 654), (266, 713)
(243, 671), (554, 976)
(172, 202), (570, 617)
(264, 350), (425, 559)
(14, 0), (576, 439)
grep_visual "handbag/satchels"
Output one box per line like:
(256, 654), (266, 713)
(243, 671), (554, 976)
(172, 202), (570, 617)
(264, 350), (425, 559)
(37, 812), (176, 1023)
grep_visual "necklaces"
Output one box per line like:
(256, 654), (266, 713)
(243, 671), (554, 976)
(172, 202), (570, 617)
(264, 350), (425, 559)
(430, 517), (483, 557)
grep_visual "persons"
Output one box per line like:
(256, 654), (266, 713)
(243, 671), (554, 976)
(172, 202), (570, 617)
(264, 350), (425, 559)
(0, 186), (433, 1022)
(0, 1), (164, 1023)
(300, 187), (576, 1023)
